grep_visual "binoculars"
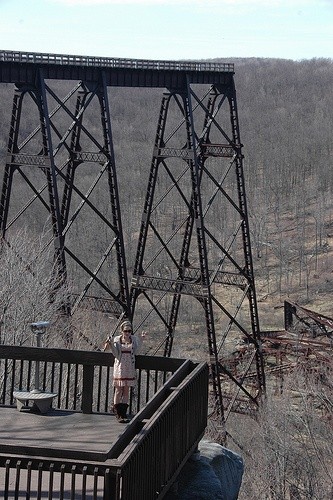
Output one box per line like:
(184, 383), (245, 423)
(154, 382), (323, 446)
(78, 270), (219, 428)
(30, 321), (50, 332)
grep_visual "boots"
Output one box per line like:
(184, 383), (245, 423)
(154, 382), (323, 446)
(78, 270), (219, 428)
(113, 403), (124, 423)
(121, 403), (131, 422)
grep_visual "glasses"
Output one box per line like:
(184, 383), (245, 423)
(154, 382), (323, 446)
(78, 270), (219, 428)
(122, 329), (132, 333)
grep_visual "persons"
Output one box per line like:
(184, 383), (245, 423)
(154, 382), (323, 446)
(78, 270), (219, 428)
(105, 321), (141, 422)
